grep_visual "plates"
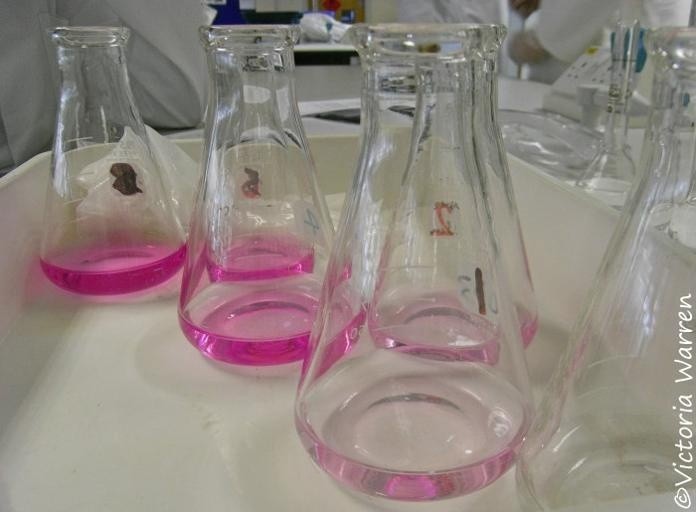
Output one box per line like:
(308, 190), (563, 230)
(0, 132), (696, 512)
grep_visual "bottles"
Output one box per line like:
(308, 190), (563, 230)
(574, 24), (645, 210)
(512, 27), (696, 512)
(370, 19), (538, 366)
(202, 30), (316, 282)
(39, 25), (186, 296)
(294, 23), (533, 500)
(177, 25), (337, 367)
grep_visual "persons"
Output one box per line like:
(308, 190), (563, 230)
(507, 0), (692, 85)
(0, 1), (209, 168)
